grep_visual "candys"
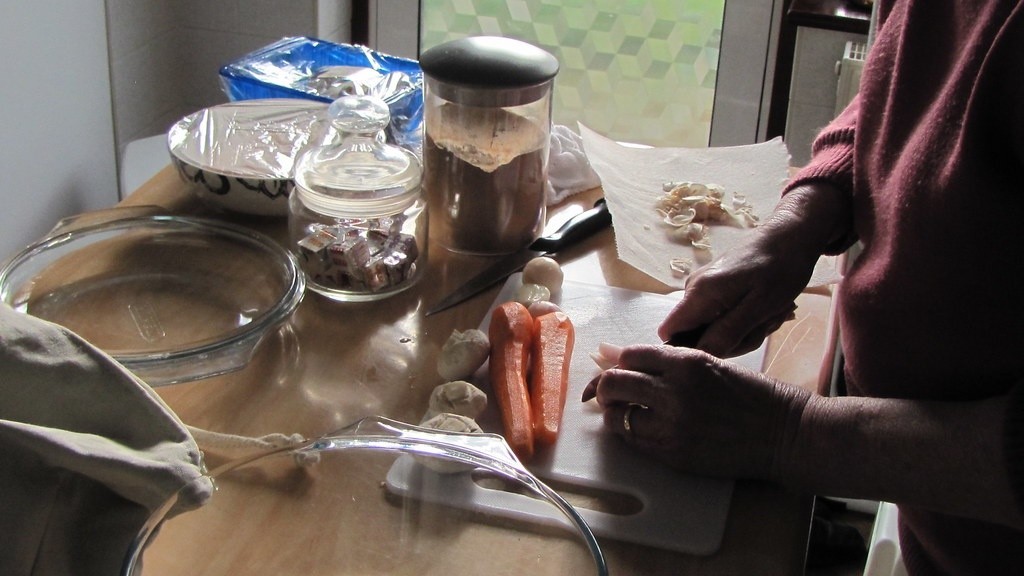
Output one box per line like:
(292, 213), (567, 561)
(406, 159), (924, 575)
(299, 211), (420, 291)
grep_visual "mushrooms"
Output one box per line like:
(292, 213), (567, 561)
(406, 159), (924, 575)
(413, 413), (485, 473)
(428, 380), (488, 421)
(436, 328), (490, 381)
(522, 257), (563, 294)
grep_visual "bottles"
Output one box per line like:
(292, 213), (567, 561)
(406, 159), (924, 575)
(287, 96), (422, 303)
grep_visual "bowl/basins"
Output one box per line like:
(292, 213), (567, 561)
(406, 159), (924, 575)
(122, 415), (607, 576)
(219, 35), (423, 132)
(0, 204), (305, 389)
(166, 100), (331, 217)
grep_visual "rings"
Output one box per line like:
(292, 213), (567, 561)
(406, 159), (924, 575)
(623, 407), (638, 432)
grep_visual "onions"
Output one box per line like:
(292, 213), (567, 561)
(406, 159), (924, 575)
(518, 283), (551, 308)
(588, 342), (625, 372)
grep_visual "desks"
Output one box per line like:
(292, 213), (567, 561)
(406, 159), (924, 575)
(11, 163), (846, 576)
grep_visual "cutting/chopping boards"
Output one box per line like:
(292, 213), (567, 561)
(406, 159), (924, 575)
(387, 272), (768, 554)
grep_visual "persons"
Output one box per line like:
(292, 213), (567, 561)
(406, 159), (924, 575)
(599, 0), (1024, 576)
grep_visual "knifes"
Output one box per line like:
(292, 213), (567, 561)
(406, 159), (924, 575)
(426, 195), (611, 316)
(582, 303), (797, 403)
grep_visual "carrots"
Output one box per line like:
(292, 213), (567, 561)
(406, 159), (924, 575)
(489, 301), (534, 454)
(528, 312), (575, 445)
